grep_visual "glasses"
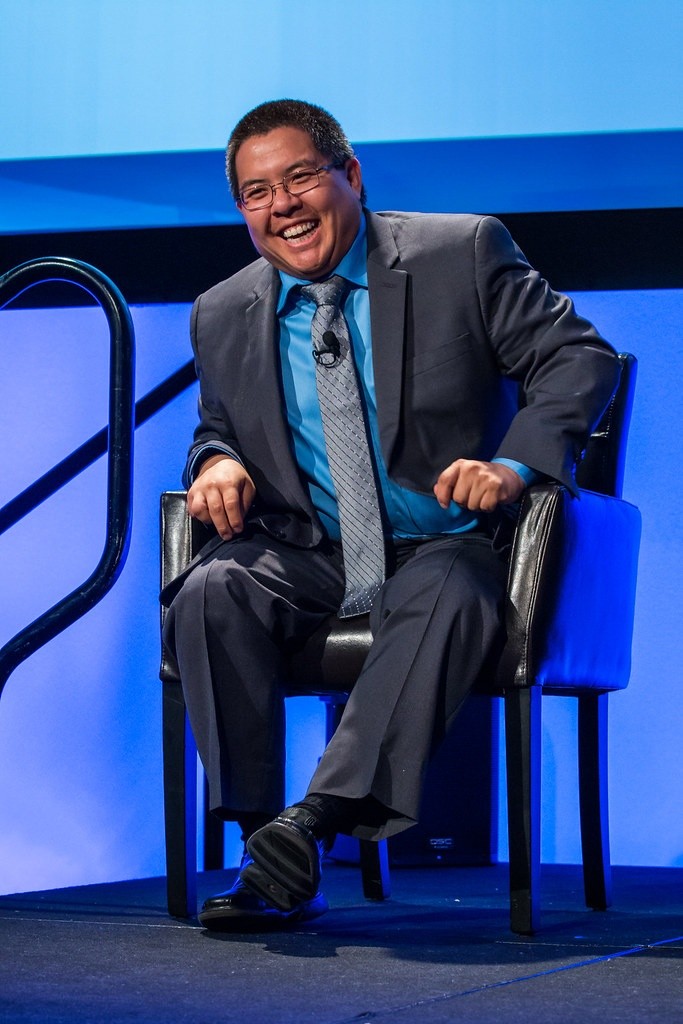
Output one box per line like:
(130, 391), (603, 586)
(235, 163), (335, 211)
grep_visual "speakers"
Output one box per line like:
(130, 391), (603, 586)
(323, 693), (497, 868)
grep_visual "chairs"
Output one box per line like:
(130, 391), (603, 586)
(159, 354), (641, 933)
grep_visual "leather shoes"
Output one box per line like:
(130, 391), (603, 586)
(241, 806), (335, 914)
(197, 858), (328, 930)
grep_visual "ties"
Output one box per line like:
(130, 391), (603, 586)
(298, 275), (385, 617)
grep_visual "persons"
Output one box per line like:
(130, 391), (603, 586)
(160, 98), (622, 925)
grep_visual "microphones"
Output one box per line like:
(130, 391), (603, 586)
(323, 330), (340, 356)
